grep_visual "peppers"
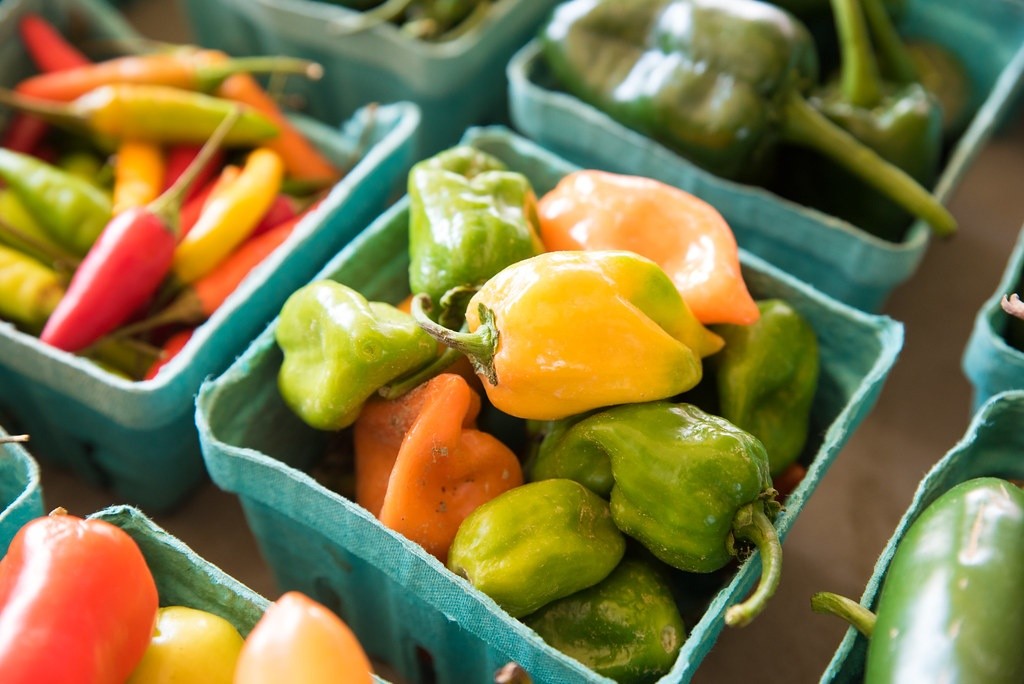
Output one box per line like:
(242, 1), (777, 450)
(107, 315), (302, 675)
(0, 0), (1024, 684)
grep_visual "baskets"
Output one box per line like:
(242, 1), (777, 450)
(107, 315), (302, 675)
(0, 1), (1022, 684)
